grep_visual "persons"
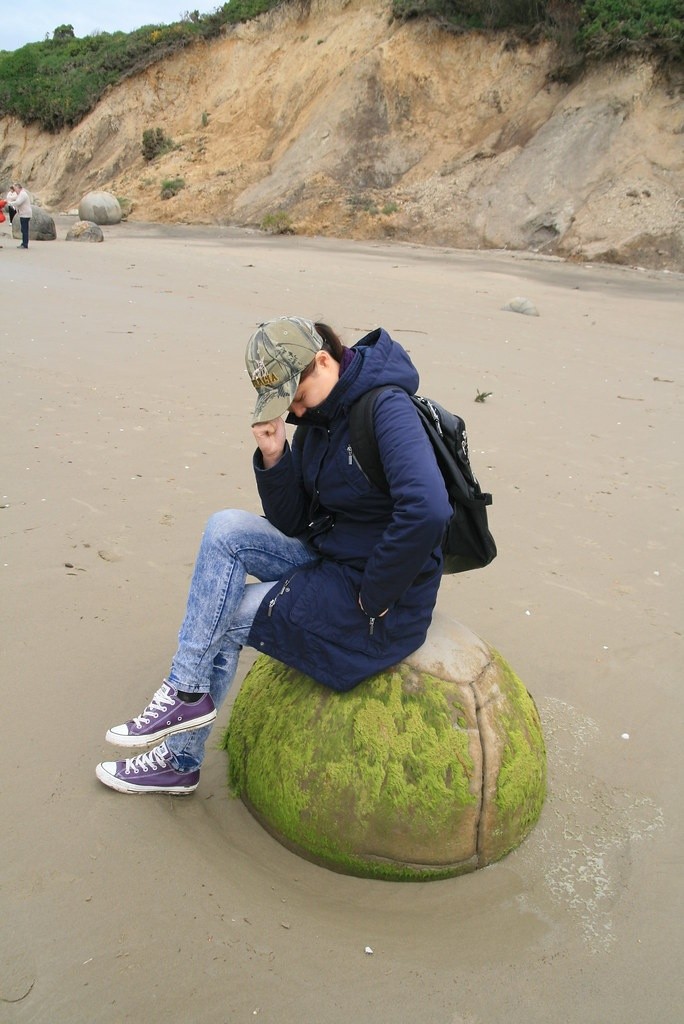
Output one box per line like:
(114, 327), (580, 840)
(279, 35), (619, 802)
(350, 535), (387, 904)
(7, 186), (17, 225)
(94, 315), (455, 794)
(0, 189), (7, 249)
(8, 184), (31, 249)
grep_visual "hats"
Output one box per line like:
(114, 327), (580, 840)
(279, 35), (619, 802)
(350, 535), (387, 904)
(245, 315), (324, 429)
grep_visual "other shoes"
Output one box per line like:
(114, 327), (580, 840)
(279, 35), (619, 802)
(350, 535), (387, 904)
(17, 244), (28, 250)
(9, 223), (12, 226)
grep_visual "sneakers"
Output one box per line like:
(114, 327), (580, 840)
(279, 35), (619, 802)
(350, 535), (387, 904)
(105, 678), (218, 748)
(95, 741), (201, 795)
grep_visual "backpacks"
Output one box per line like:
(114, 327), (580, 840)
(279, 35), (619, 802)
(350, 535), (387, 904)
(348, 383), (498, 576)
